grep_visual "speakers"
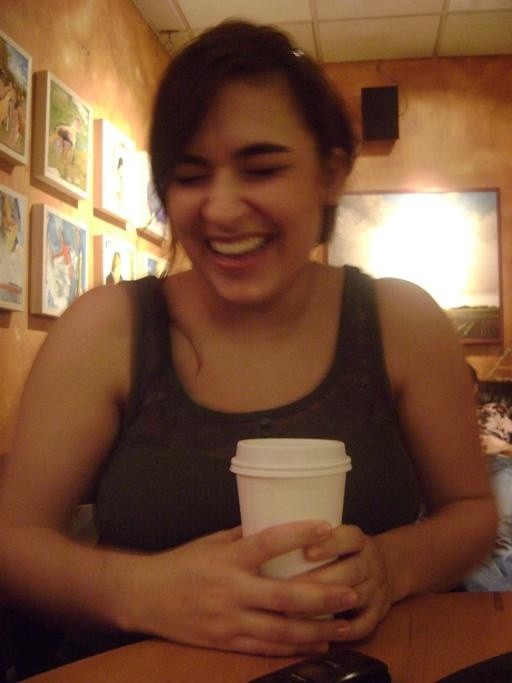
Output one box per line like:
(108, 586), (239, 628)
(361, 85), (399, 140)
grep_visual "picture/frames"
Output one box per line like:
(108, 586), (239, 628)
(96, 121), (168, 244)
(93, 234), (168, 285)
(1, 186), (32, 312)
(32, 201), (90, 323)
(35, 68), (95, 196)
(1, 29), (35, 168)
(322, 188), (504, 347)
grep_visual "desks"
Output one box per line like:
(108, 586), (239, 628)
(12, 580), (512, 683)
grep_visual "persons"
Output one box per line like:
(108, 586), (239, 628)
(1, 19), (499, 658)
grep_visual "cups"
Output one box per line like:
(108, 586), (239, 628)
(228, 437), (352, 623)
